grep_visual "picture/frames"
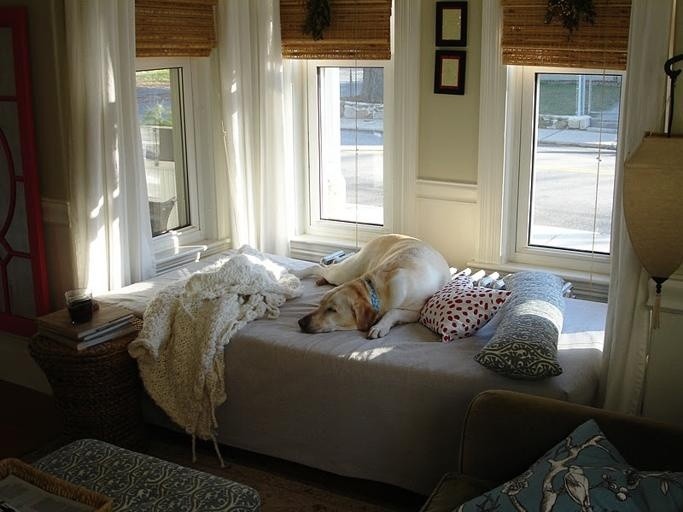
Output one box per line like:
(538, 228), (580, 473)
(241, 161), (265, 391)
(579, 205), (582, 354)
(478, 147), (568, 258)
(433, 1), (467, 95)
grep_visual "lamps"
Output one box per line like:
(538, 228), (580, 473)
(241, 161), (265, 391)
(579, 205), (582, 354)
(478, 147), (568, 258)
(621, 52), (683, 331)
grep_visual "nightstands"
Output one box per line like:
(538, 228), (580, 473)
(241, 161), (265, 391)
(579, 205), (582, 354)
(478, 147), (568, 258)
(27, 319), (151, 459)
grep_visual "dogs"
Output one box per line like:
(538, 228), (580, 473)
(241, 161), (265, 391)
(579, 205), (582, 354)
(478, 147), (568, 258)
(288, 233), (453, 340)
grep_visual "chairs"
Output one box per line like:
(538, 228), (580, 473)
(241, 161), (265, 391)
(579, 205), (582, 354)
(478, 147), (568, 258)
(26, 436), (263, 512)
(413, 389), (682, 511)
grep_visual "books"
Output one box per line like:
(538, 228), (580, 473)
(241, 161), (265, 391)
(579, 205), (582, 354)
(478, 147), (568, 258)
(37, 297), (133, 336)
(35, 322), (136, 352)
(34, 312), (137, 341)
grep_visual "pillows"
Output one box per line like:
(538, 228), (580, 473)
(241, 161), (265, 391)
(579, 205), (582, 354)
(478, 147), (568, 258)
(420, 270), (567, 381)
(447, 418), (683, 512)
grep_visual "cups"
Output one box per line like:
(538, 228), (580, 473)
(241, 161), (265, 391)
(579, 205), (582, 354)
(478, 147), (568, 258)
(63, 288), (93, 323)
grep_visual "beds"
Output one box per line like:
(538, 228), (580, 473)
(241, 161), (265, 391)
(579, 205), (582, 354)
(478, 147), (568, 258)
(91, 247), (608, 501)
